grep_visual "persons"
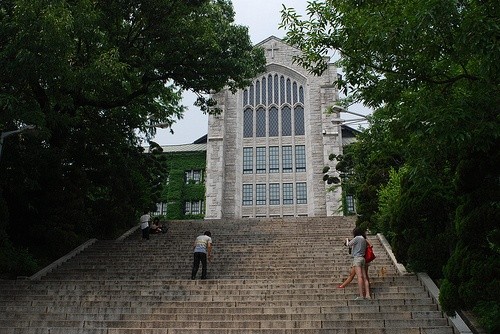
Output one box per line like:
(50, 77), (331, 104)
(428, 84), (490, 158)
(140, 210), (163, 241)
(191, 230), (213, 280)
(338, 226), (373, 300)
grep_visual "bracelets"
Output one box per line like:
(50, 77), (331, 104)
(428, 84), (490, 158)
(208, 255), (210, 256)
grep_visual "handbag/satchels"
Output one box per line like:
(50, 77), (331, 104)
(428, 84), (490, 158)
(364, 239), (377, 263)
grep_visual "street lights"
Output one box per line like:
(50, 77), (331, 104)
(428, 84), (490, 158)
(331, 105), (371, 127)
(0, 125), (39, 155)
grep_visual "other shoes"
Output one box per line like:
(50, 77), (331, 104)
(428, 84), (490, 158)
(354, 296), (364, 301)
(337, 285), (346, 288)
(362, 295), (371, 299)
(201, 277), (208, 279)
(191, 276), (195, 280)
